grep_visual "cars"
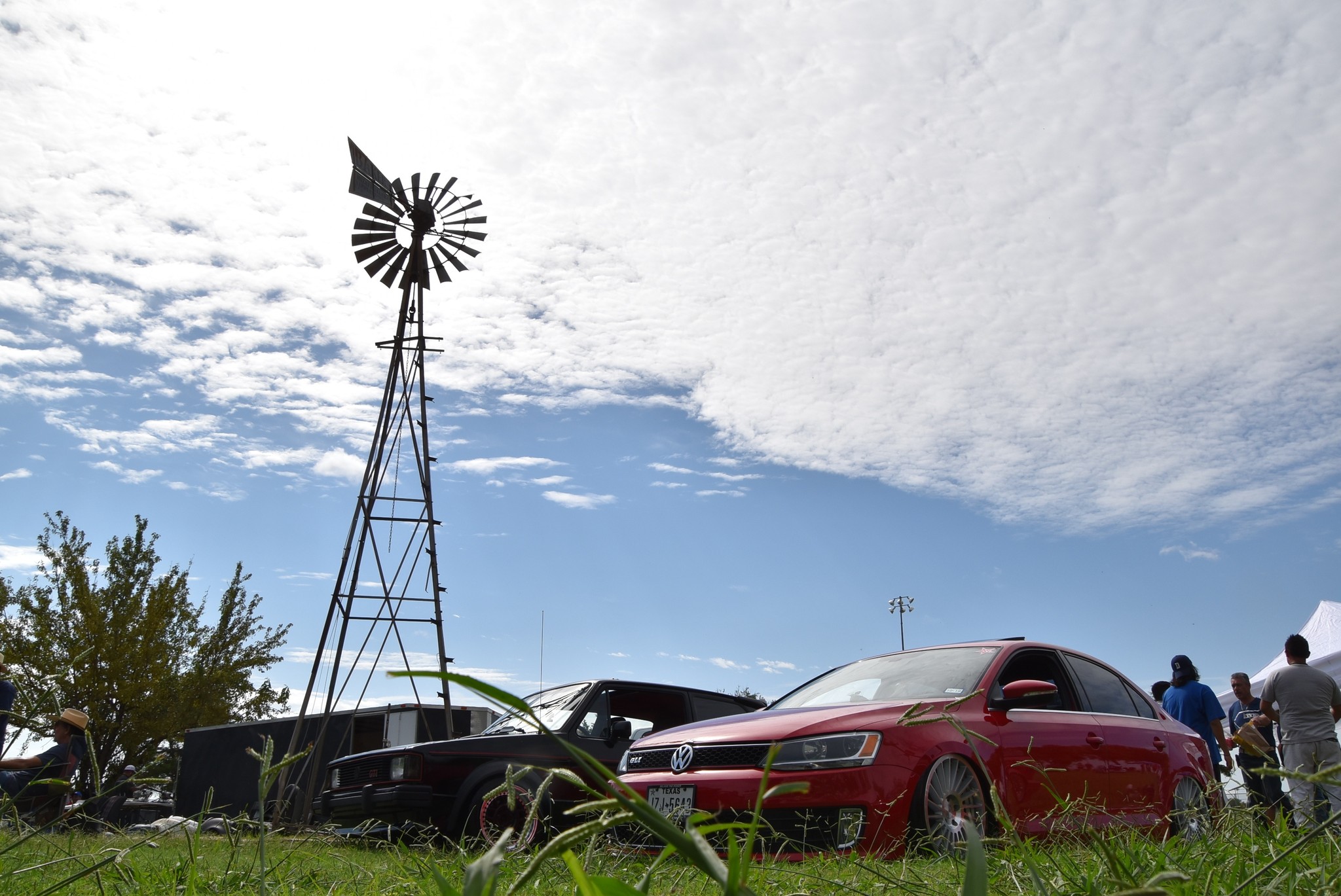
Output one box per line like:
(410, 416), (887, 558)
(606, 636), (1230, 865)
(320, 680), (768, 857)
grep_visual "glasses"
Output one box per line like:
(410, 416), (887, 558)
(54, 721), (67, 729)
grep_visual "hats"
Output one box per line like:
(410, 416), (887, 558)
(45, 708), (90, 732)
(0, 652), (8, 673)
(1171, 655), (1193, 679)
(124, 765), (138, 774)
(72, 791), (82, 798)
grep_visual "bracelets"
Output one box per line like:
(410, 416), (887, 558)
(1223, 753), (1231, 756)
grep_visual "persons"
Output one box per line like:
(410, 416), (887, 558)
(114, 765), (148, 798)
(1161, 655), (1234, 808)
(1, 706), (91, 799)
(67, 791), (82, 804)
(1259, 633), (1341, 830)
(1225, 673), (1299, 836)
(1150, 681), (1172, 707)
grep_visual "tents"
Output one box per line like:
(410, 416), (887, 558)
(1215, 601), (1341, 804)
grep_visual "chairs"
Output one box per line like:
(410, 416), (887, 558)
(1027, 688), (1072, 711)
(67, 795), (127, 834)
(0, 733), (88, 836)
(600, 717), (629, 739)
